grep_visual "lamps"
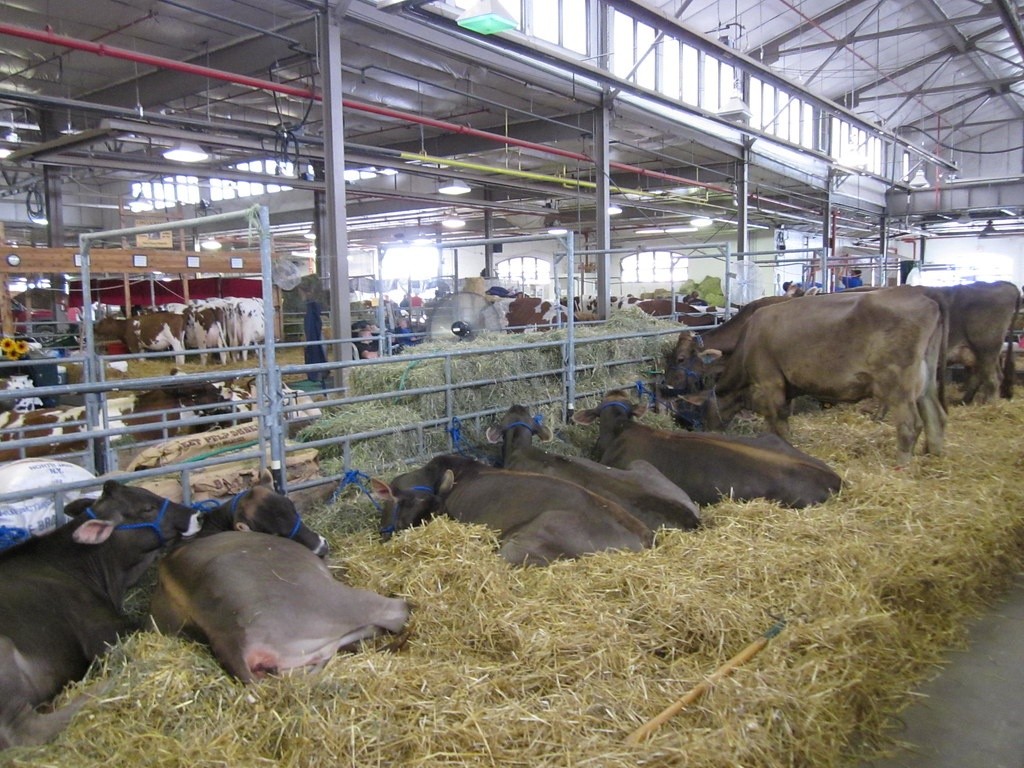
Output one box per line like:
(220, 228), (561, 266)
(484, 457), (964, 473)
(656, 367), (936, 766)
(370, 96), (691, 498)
(200, 235), (222, 251)
(908, 169), (930, 188)
(955, 214), (995, 239)
(715, 90), (753, 123)
(160, 141), (208, 164)
(443, 210), (465, 228)
(690, 216), (713, 226)
(609, 204), (621, 213)
(548, 221), (566, 235)
(453, 0), (518, 35)
(436, 179), (470, 196)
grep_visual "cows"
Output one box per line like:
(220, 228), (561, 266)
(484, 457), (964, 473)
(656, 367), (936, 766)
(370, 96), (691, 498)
(0, 366), (321, 458)
(0, 481), (205, 755)
(145, 474), (412, 685)
(93, 293), (265, 368)
(674, 285), (950, 472)
(659, 280), (1021, 407)
(492, 288), (739, 335)
(380, 385), (848, 567)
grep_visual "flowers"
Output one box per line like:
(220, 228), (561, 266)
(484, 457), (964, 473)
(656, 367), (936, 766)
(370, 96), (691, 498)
(0, 338), (30, 360)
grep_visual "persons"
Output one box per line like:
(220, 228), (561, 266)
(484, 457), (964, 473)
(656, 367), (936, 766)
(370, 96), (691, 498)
(400, 293), (421, 307)
(65, 306), (82, 346)
(351, 321), (395, 359)
(838, 266), (863, 288)
(783, 281), (801, 297)
(394, 317), (417, 349)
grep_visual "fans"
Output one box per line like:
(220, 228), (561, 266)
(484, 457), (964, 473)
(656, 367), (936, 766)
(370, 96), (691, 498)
(720, 261), (766, 307)
(426, 290), (502, 345)
